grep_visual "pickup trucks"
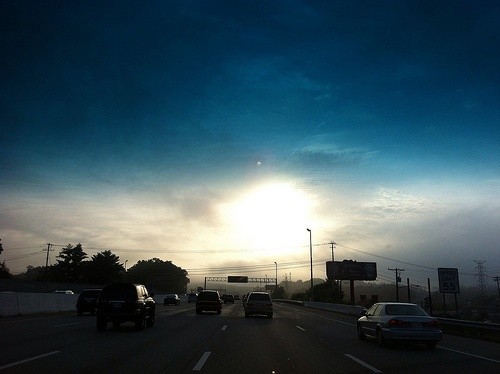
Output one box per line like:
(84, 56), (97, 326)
(194, 289), (223, 315)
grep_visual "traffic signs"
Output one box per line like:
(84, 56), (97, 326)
(227, 276), (248, 283)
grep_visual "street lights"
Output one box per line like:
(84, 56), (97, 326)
(274, 262), (278, 299)
(307, 228), (314, 301)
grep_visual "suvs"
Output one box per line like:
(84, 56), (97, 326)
(96, 282), (157, 331)
(53, 289), (75, 294)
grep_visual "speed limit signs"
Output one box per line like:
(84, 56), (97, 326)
(437, 267), (460, 294)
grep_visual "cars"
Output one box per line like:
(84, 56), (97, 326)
(234, 294), (241, 300)
(223, 294), (236, 304)
(163, 293), (181, 306)
(355, 300), (445, 349)
(187, 291), (201, 304)
(75, 289), (103, 316)
(243, 291), (274, 319)
